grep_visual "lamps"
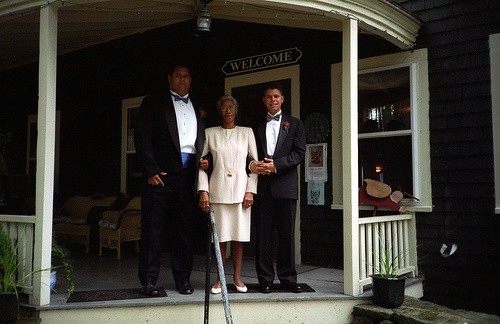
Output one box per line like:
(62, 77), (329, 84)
(195, 0), (213, 32)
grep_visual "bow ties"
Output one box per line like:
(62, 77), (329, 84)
(266, 113), (282, 122)
(170, 91), (191, 104)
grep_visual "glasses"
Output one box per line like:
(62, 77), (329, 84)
(219, 105), (237, 112)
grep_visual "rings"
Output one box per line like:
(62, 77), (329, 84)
(249, 204), (251, 205)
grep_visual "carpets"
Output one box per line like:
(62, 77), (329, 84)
(192, 257), (319, 278)
(66, 287), (168, 303)
(227, 283), (317, 293)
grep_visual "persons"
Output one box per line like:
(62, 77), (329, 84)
(197, 96), (259, 294)
(133, 62), (213, 295)
(246, 86), (306, 293)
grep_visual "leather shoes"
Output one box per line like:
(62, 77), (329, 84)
(174, 281), (194, 294)
(232, 281), (248, 293)
(258, 283), (272, 293)
(282, 283), (303, 293)
(210, 283), (222, 294)
(141, 284), (167, 297)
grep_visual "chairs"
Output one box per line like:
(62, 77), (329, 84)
(98, 195), (144, 261)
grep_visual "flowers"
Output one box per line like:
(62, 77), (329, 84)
(283, 122), (291, 130)
(199, 108), (207, 120)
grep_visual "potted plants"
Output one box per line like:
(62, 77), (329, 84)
(0, 237), (74, 324)
(358, 230), (429, 311)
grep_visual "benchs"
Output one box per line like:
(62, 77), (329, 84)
(55, 194), (122, 254)
